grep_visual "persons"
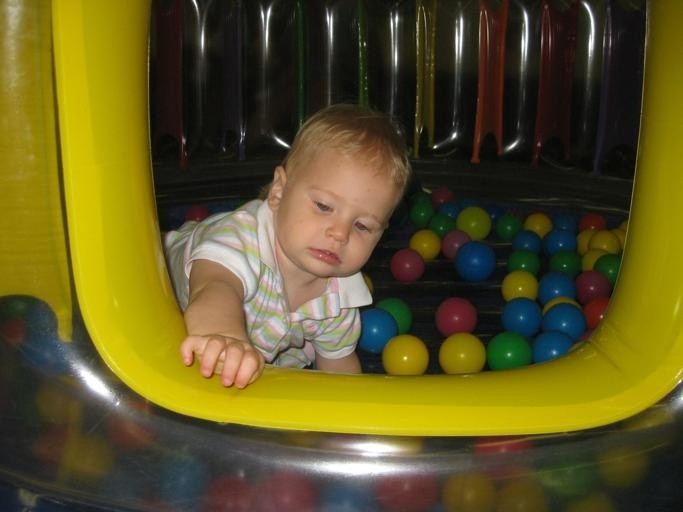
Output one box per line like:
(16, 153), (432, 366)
(160, 102), (416, 390)
